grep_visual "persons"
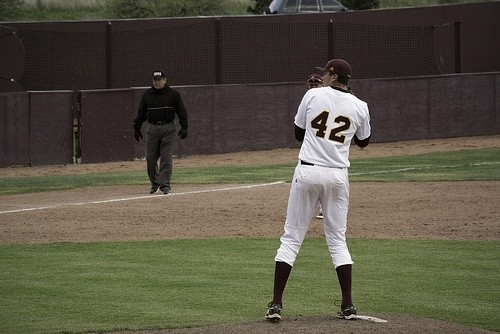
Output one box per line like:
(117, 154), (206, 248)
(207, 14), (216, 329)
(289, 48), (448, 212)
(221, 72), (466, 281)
(133, 70), (189, 194)
(264, 59), (371, 322)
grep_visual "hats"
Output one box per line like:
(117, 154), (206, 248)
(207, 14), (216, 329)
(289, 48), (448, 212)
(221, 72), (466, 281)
(315, 59), (352, 78)
(152, 71), (166, 80)
(306, 73), (322, 84)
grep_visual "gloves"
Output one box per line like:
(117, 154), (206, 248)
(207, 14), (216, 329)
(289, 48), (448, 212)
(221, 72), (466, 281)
(133, 123), (143, 142)
(178, 125), (188, 140)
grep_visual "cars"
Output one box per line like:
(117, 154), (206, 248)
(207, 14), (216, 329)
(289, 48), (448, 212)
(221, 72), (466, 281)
(264, 0), (349, 14)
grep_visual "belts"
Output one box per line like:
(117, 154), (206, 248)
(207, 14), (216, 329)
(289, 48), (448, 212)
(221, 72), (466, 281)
(301, 160), (314, 166)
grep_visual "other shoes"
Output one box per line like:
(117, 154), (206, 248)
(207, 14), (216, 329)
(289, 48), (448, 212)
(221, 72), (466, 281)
(162, 187), (170, 195)
(150, 186), (160, 194)
(334, 300), (357, 320)
(264, 302), (282, 320)
